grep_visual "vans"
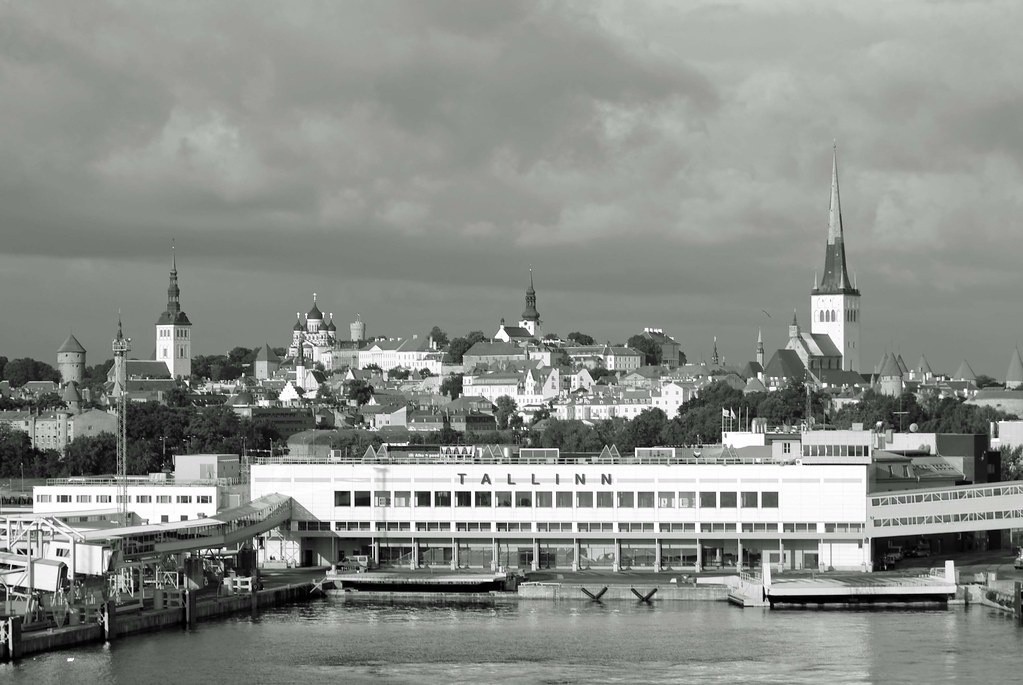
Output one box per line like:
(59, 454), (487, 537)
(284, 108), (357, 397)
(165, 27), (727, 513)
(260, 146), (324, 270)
(336, 555), (369, 570)
